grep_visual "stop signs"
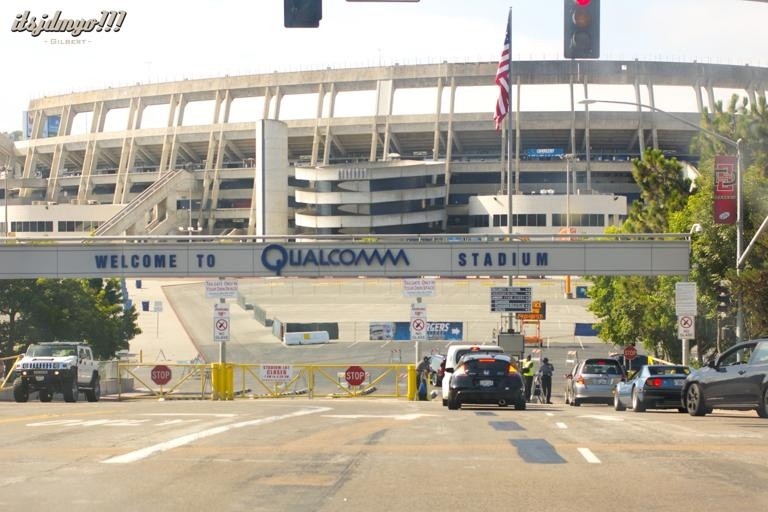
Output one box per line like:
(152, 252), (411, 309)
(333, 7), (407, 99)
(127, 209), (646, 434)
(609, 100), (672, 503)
(622, 346), (637, 360)
(151, 365), (171, 385)
(344, 366), (365, 386)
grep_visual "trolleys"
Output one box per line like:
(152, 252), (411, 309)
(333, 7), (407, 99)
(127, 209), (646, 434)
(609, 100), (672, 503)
(521, 318), (544, 346)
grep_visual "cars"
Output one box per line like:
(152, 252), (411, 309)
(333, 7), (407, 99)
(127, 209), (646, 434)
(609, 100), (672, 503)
(606, 352), (652, 372)
(442, 351), (528, 413)
(425, 354), (445, 385)
(677, 338), (767, 420)
(562, 355), (625, 408)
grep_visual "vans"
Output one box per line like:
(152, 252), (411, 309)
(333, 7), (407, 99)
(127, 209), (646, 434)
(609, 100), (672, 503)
(439, 342), (503, 409)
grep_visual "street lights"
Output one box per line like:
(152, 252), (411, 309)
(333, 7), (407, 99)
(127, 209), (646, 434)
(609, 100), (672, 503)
(0, 166), (13, 244)
(576, 96), (746, 364)
(557, 153), (584, 241)
(176, 224), (203, 242)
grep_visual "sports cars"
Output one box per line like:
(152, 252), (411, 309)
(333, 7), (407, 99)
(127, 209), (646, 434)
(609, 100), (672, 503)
(610, 364), (713, 413)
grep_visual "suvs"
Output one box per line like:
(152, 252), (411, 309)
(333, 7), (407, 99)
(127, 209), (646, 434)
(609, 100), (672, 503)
(8, 339), (104, 403)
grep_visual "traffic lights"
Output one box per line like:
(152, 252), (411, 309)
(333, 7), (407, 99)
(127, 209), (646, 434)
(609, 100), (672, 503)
(563, 0), (601, 59)
(284, 0), (322, 28)
(715, 285), (729, 312)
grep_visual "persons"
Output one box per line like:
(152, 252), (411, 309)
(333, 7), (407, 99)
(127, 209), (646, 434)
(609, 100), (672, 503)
(521, 355), (534, 400)
(440, 361), (448, 376)
(416, 356), (433, 389)
(540, 358), (554, 404)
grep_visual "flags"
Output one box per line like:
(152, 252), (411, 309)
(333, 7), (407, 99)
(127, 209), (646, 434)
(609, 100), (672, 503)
(494, 11), (514, 131)
